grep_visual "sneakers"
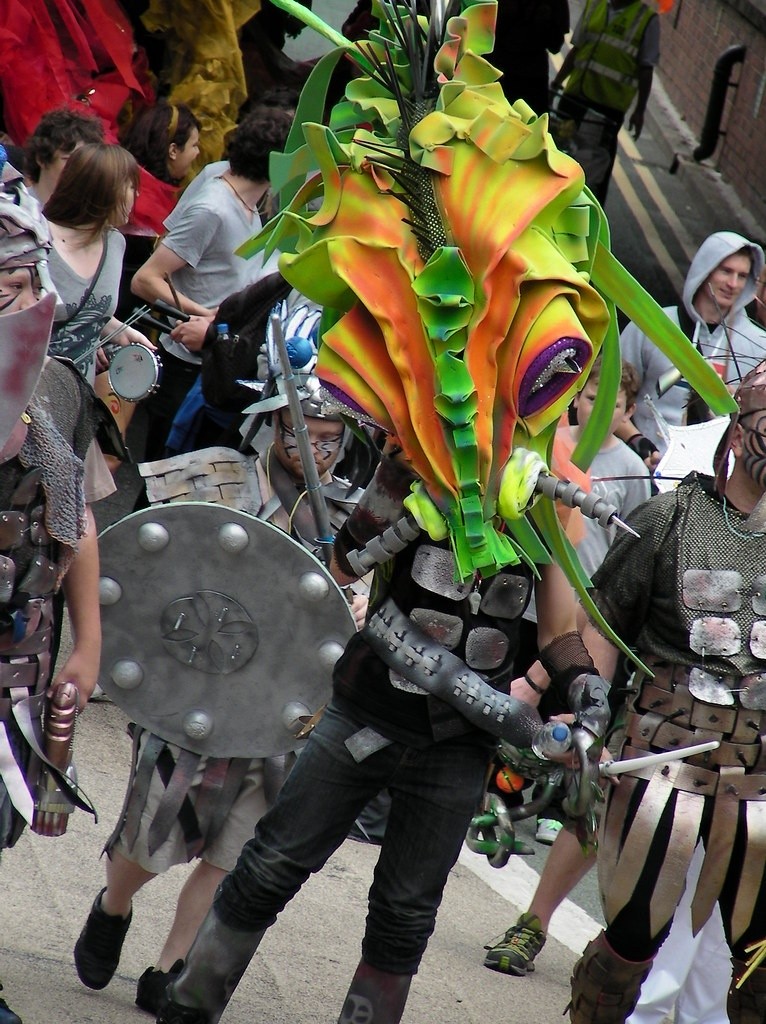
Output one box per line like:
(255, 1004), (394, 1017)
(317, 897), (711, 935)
(483, 912), (546, 976)
(135, 958), (184, 1014)
(536, 818), (563, 844)
(74, 887), (133, 990)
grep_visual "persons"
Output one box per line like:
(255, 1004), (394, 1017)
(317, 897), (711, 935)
(0, 0), (766, 1024)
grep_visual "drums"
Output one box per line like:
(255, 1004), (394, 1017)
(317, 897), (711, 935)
(108, 342), (164, 404)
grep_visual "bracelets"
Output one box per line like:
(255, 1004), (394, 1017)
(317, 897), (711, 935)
(525, 674), (547, 694)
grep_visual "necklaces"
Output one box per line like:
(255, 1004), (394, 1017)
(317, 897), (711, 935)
(221, 176), (270, 216)
(723, 496), (765, 538)
(21, 412), (32, 424)
